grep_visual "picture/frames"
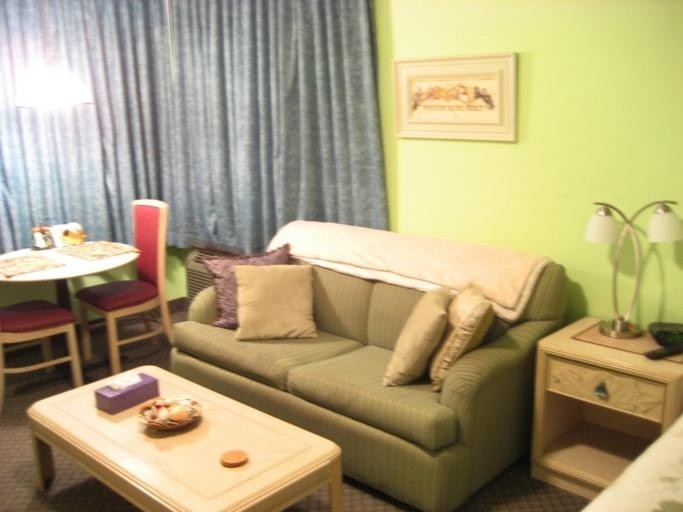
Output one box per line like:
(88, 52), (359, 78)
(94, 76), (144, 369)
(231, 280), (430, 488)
(394, 52), (517, 143)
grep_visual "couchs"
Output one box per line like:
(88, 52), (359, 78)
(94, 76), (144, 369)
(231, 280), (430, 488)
(169, 219), (565, 512)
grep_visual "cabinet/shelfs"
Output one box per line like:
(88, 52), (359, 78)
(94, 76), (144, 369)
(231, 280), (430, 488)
(531, 315), (683, 500)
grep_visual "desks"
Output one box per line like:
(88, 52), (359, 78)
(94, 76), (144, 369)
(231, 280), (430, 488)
(0, 242), (141, 312)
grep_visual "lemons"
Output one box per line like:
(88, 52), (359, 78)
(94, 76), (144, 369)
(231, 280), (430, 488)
(169, 405), (189, 421)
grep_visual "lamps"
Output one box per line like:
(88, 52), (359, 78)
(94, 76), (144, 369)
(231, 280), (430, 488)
(584, 200), (683, 340)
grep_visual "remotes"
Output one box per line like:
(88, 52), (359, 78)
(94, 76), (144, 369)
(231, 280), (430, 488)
(644, 343), (683, 359)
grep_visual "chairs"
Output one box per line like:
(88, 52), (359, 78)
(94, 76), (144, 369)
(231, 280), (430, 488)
(0, 300), (85, 411)
(75, 199), (174, 376)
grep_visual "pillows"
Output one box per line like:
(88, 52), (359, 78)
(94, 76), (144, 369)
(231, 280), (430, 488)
(428, 276), (493, 392)
(382, 286), (450, 387)
(232, 264), (319, 341)
(202, 243), (291, 329)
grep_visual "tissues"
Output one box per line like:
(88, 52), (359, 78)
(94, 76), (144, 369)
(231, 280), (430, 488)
(95, 368), (157, 414)
